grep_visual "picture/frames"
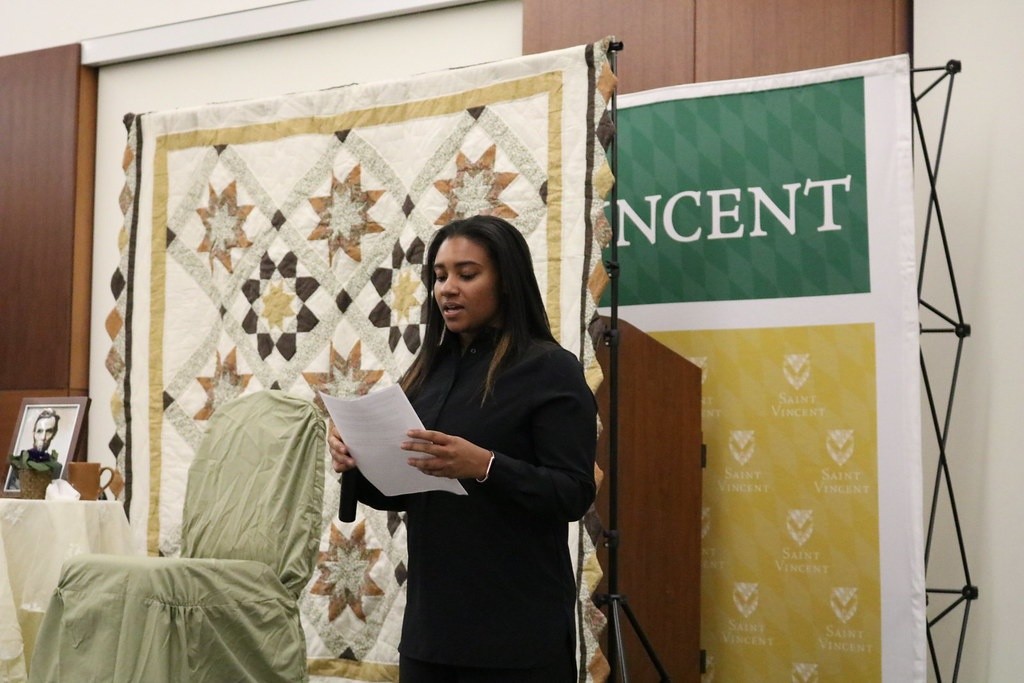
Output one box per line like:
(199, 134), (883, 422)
(0, 396), (93, 500)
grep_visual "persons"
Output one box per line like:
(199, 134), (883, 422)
(324, 215), (600, 681)
(7, 409), (64, 490)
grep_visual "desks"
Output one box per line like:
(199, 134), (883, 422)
(58, 387), (330, 681)
(0, 497), (137, 682)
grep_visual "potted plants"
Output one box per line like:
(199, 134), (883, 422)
(8, 449), (64, 500)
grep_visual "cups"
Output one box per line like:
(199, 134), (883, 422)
(68, 460), (114, 502)
